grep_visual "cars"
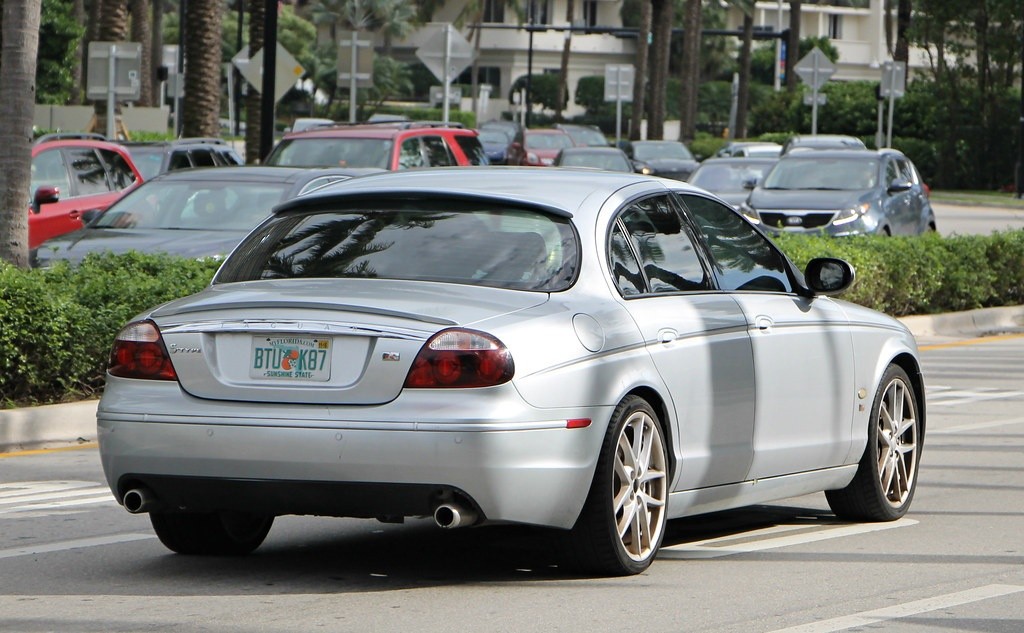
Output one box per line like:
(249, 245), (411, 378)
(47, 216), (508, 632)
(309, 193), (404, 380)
(90, 161), (930, 576)
(28, 166), (388, 272)
(781, 134), (868, 157)
(116, 137), (244, 187)
(477, 119), (784, 209)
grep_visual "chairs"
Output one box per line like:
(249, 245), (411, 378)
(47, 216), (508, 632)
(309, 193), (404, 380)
(191, 193), (224, 227)
(477, 229), (544, 292)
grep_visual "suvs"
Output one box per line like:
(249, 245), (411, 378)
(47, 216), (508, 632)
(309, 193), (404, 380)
(28, 130), (145, 253)
(736, 149), (938, 242)
(263, 120), (491, 170)
